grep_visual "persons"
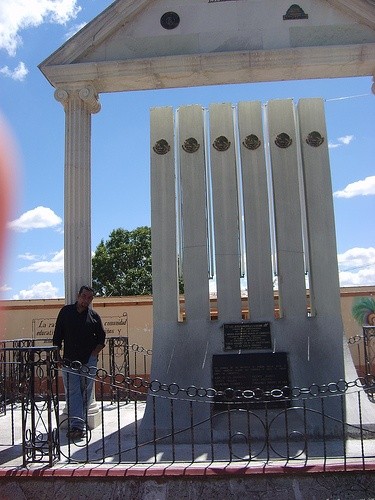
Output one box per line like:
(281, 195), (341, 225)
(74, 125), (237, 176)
(51, 286), (108, 440)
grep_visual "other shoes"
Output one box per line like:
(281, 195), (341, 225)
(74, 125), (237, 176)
(67, 428), (86, 439)
